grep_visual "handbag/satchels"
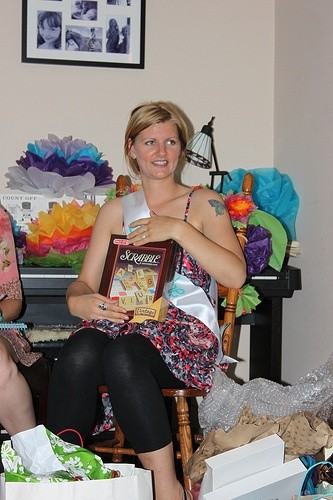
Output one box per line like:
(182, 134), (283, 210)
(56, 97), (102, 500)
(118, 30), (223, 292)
(0, 423), (152, 500)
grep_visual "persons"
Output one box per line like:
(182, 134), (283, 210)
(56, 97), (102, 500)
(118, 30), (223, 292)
(0, 205), (43, 437)
(37, 11), (62, 50)
(65, 0), (130, 53)
(46, 101), (248, 500)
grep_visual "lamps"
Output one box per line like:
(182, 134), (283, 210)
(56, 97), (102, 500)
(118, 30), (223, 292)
(185, 116), (233, 194)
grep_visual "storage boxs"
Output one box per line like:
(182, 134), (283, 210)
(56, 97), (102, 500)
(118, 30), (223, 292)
(99, 233), (178, 324)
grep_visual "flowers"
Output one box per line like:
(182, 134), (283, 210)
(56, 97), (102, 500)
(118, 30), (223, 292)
(5, 135), (117, 202)
(223, 192), (257, 229)
(24, 198), (103, 273)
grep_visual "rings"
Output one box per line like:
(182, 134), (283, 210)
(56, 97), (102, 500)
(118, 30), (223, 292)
(99, 302), (108, 311)
(142, 233), (146, 238)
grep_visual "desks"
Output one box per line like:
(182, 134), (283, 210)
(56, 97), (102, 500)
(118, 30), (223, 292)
(16, 263), (302, 387)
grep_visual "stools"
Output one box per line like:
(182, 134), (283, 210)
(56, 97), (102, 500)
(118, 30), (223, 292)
(0, 356), (50, 458)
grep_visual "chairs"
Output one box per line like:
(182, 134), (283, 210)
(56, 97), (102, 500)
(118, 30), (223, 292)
(86, 174), (252, 500)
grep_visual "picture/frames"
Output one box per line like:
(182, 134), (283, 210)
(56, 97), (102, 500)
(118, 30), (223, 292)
(20, 0), (145, 70)
